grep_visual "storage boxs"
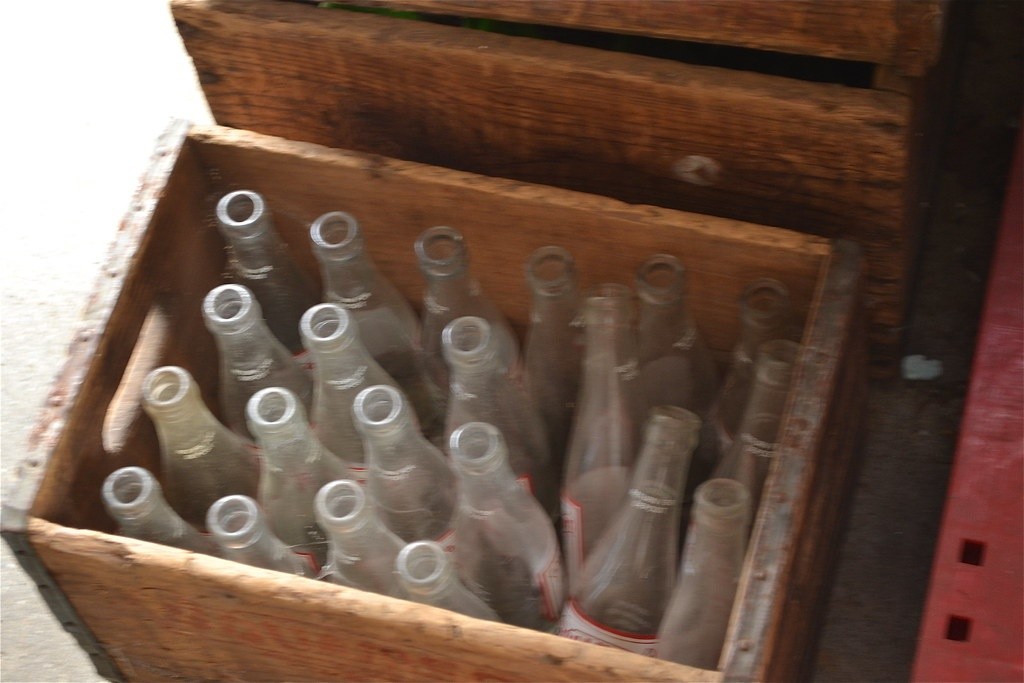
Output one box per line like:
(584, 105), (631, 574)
(169, 1), (956, 403)
(0, 118), (870, 682)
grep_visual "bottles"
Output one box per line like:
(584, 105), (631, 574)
(630, 251), (721, 409)
(206, 494), (315, 589)
(142, 363), (258, 524)
(442, 315), (560, 532)
(242, 386), (355, 572)
(654, 477), (752, 669)
(554, 403), (698, 658)
(693, 277), (802, 470)
(562, 281), (653, 606)
(525, 247), (580, 459)
(214, 191), (320, 379)
(100, 464), (213, 566)
(297, 300), (421, 489)
(444, 420), (565, 630)
(202, 284), (315, 455)
(706, 340), (803, 510)
(353, 382), (466, 573)
(313, 474), (406, 601)
(392, 538), (500, 624)
(310, 209), (434, 429)
(412, 226), (525, 429)
